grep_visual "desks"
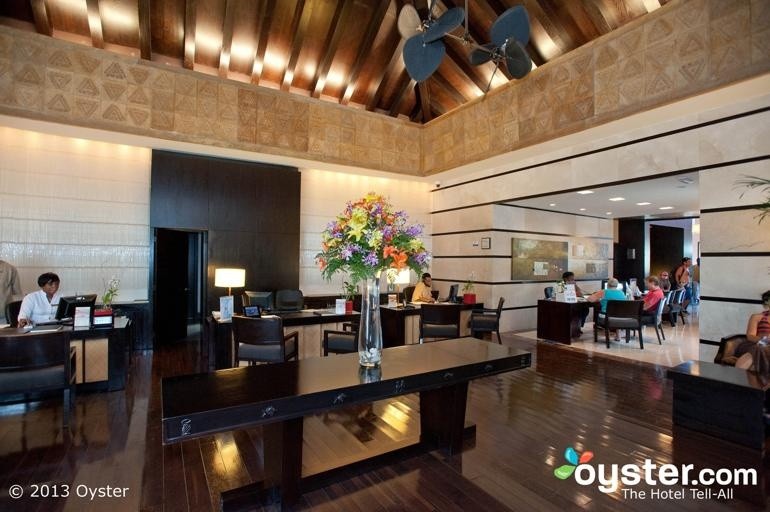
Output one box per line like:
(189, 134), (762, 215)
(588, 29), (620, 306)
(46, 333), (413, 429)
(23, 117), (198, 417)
(664, 358), (770, 452)
(159, 335), (532, 511)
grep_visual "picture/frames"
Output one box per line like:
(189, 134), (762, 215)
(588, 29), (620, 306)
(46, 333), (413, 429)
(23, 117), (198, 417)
(480, 238), (491, 248)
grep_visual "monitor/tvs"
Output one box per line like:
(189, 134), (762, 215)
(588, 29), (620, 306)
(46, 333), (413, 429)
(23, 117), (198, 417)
(55, 294), (97, 319)
(448, 284), (459, 303)
(602, 279), (626, 295)
(245, 291), (273, 310)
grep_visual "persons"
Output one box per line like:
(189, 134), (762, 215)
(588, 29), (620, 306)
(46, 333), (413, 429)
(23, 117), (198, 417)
(734, 289), (770, 370)
(15, 272), (64, 329)
(552, 255), (700, 344)
(0, 260), (24, 325)
(411, 273), (435, 302)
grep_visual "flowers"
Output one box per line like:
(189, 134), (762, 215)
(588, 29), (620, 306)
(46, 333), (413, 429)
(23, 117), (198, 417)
(313, 191), (432, 283)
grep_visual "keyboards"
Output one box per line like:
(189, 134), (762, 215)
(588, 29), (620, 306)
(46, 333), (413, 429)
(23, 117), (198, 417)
(36, 318), (71, 326)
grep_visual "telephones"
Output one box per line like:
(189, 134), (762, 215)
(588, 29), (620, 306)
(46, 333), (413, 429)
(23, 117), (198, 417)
(403, 299), (415, 308)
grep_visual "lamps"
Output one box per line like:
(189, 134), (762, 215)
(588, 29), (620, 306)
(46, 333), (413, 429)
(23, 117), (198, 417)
(214, 268), (246, 296)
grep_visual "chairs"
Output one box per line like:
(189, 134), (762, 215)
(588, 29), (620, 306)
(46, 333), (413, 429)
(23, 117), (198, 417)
(2, 300), (150, 429)
(526, 276), (687, 350)
(716, 334), (747, 365)
(208, 284), (505, 370)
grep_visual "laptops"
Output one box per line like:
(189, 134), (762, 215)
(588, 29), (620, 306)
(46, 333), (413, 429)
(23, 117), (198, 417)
(244, 306), (261, 318)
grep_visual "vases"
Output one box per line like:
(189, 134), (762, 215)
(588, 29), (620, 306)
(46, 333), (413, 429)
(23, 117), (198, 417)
(357, 277), (383, 367)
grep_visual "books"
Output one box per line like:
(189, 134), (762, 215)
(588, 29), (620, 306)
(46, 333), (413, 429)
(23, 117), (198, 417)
(313, 310), (337, 316)
(407, 303), (422, 309)
(28, 324), (63, 332)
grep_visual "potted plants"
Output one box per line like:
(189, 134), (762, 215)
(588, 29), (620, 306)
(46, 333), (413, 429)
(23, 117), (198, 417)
(462, 280), (477, 304)
(341, 281), (356, 313)
